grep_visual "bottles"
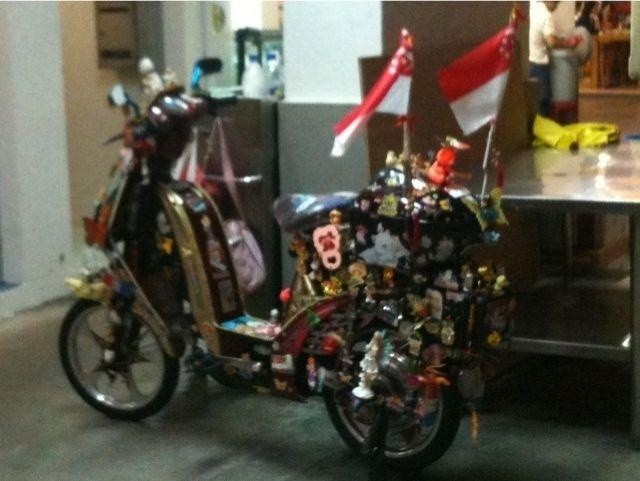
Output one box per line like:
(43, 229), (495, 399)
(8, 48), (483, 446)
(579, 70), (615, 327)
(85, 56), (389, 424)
(241, 53), (286, 102)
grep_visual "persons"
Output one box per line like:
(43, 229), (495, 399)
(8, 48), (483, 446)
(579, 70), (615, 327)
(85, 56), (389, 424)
(575, 2), (640, 88)
(528, 1), (577, 118)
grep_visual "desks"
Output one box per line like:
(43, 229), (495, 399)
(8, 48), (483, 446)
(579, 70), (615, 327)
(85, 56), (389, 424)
(469, 138), (640, 442)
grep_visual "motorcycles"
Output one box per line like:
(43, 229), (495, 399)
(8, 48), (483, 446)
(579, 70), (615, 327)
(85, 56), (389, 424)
(60, 58), (513, 473)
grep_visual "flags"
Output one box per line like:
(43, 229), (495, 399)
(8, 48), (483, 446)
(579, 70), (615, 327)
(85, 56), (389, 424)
(327, 28), (416, 156)
(438, 8), (519, 140)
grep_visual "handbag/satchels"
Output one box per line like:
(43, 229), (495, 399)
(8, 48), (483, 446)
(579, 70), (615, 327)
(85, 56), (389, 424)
(207, 218), (266, 294)
